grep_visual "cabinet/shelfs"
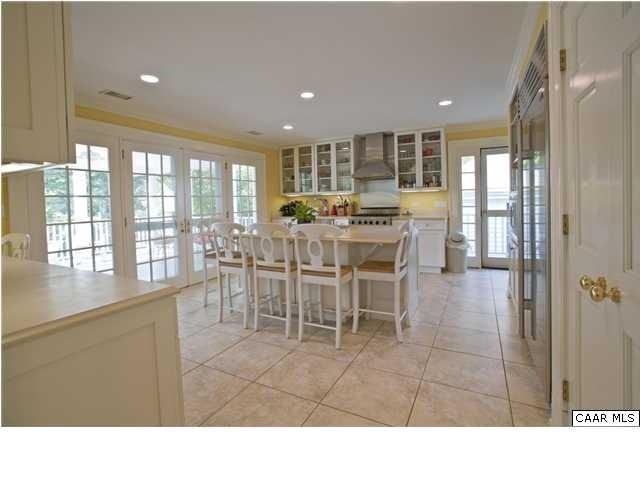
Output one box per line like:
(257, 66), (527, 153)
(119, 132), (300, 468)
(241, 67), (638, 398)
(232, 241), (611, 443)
(314, 139), (366, 195)
(415, 217), (449, 275)
(1, 252), (190, 428)
(1, 2), (80, 176)
(279, 144), (314, 197)
(394, 126), (448, 192)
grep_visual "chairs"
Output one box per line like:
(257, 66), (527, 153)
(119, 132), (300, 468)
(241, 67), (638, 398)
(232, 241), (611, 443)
(2, 232), (31, 260)
(195, 215), (419, 348)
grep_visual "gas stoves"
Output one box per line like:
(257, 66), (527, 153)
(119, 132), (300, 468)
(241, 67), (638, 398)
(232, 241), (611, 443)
(349, 213), (399, 225)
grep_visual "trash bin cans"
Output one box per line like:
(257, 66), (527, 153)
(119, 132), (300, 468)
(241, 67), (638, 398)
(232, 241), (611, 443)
(447, 233), (468, 273)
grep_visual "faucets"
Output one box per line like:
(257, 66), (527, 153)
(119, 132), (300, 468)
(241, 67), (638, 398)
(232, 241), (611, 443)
(313, 196), (329, 217)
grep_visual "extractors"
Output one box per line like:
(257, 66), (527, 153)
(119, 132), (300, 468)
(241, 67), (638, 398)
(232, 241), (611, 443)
(352, 132), (394, 180)
